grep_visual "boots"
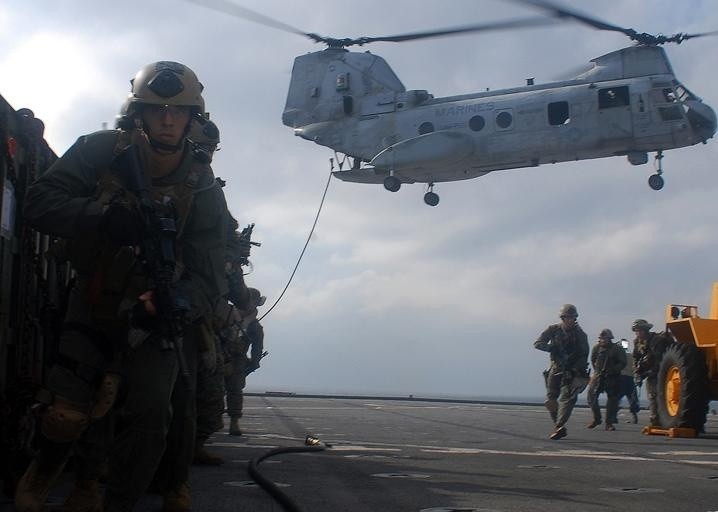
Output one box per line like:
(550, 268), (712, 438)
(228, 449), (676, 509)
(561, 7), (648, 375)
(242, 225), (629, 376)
(164, 417), (242, 511)
(550, 413), (658, 441)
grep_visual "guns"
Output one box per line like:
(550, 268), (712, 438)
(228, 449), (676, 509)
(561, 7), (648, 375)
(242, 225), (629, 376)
(591, 350), (609, 407)
(110, 143), (198, 395)
(554, 332), (576, 394)
(226, 350), (269, 384)
(239, 225), (260, 251)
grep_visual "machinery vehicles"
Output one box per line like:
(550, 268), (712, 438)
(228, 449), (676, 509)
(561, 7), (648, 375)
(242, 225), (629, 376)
(646, 282), (717, 438)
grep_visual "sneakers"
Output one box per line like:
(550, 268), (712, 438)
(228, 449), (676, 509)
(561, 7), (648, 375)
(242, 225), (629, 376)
(15, 469), (102, 512)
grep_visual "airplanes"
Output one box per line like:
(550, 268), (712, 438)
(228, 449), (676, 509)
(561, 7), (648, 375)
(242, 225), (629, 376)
(193, 0), (715, 205)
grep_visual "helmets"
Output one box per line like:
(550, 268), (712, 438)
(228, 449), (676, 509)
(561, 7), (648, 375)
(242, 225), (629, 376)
(116, 61), (221, 156)
(598, 329), (614, 339)
(632, 319), (653, 329)
(559, 304), (578, 318)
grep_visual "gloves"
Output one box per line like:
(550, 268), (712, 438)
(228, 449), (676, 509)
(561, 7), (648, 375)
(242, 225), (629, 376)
(200, 337), (218, 378)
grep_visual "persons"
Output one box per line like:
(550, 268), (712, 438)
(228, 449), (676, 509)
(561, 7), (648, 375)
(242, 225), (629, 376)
(632, 320), (674, 427)
(610, 339), (640, 424)
(214, 288), (269, 436)
(196, 232), (260, 468)
(159, 120), (221, 512)
(586, 329), (627, 431)
(15, 60), (207, 512)
(533, 304), (590, 439)
